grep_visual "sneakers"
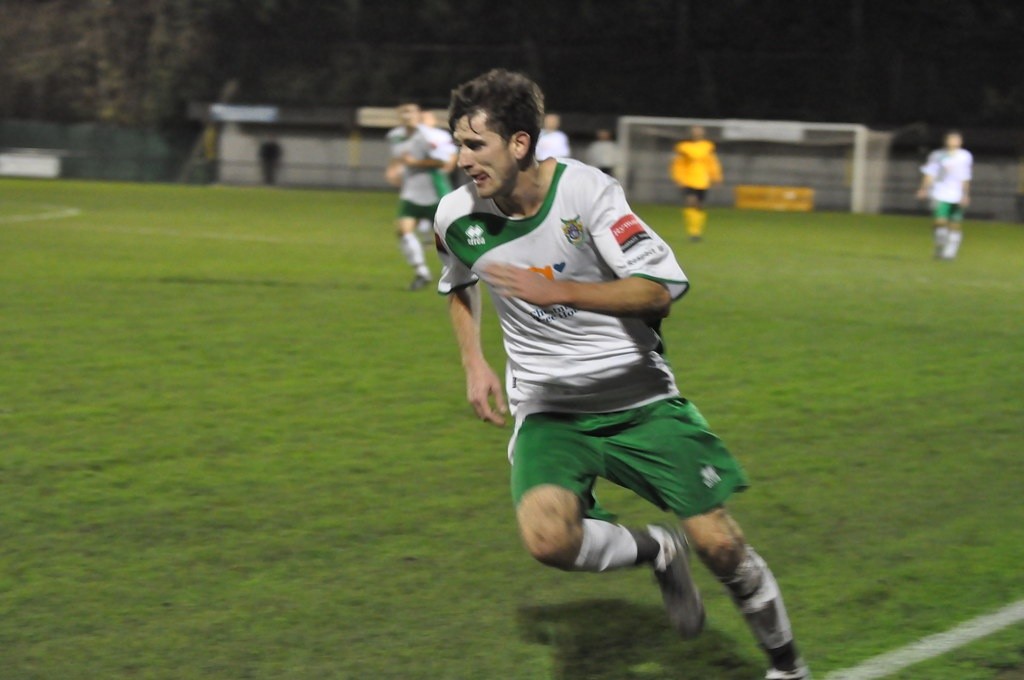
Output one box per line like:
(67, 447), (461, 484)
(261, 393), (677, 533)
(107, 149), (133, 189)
(765, 665), (810, 680)
(654, 521), (705, 639)
(411, 269), (432, 291)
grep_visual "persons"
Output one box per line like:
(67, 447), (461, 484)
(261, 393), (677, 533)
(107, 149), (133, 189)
(536, 115), (572, 165)
(588, 130), (617, 176)
(918, 133), (973, 259)
(387, 100), (458, 292)
(670, 126), (723, 241)
(433, 69), (808, 680)
